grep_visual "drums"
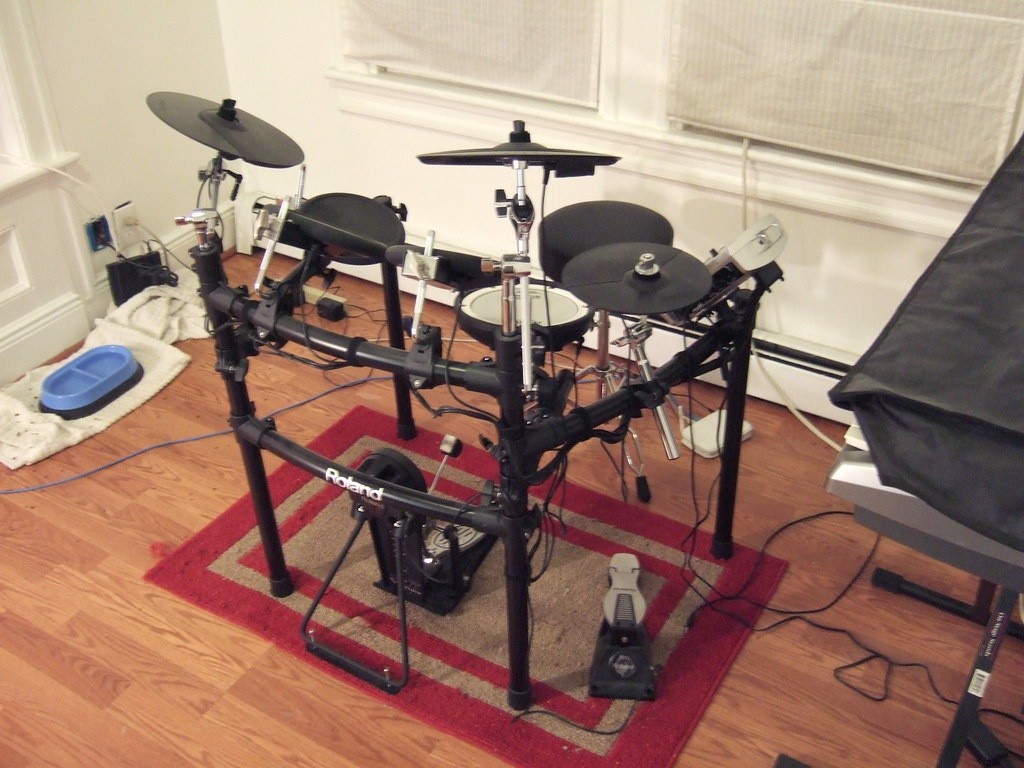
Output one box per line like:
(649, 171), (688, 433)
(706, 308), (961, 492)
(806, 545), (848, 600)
(455, 280), (596, 348)
(296, 192), (406, 266)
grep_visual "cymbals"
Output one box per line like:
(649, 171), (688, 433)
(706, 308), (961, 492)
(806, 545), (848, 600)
(146, 91), (305, 169)
(416, 141), (621, 167)
(562, 242), (714, 315)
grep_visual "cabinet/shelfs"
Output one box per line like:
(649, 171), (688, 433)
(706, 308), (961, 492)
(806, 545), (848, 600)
(2, 149), (96, 392)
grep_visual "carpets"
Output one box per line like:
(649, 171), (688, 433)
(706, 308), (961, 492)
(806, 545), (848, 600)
(139, 404), (793, 767)
